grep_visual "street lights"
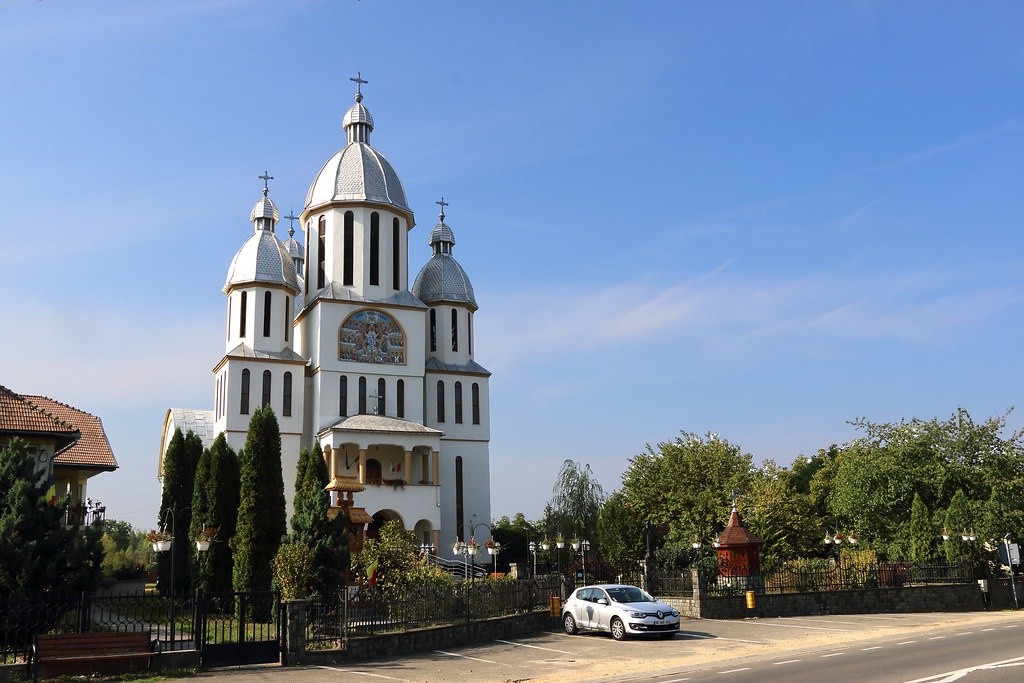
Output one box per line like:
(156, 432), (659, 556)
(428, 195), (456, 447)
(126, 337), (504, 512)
(452, 519), (497, 619)
(147, 506), (214, 650)
(460, 539), (468, 582)
(538, 524), (581, 607)
(942, 526), (977, 555)
(692, 528), (719, 562)
(420, 542), (437, 564)
(528, 541), (537, 577)
(824, 521), (859, 590)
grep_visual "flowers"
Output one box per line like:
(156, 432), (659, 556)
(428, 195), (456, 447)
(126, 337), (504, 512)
(467, 540), (476, 549)
(449, 542), (461, 551)
(849, 533), (858, 539)
(152, 529), (176, 542)
(485, 539), (496, 549)
(188, 525), (217, 543)
(833, 533), (844, 540)
(147, 533), (158, 543)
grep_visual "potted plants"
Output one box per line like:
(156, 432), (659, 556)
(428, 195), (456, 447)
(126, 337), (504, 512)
(941, 528), (950, 541)
(970, 532), (975, 541)
(962, 529), (970, 540)
(556, 537), (565, 548)
(823, 534), (833, 544)
(570, 538), (581, 549)
(713, 538), (720, 547)
(542, 539), (550, 550)
(690, 534), (701, 548)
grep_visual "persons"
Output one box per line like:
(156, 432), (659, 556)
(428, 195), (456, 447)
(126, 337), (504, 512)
(978, 571), (992, 610)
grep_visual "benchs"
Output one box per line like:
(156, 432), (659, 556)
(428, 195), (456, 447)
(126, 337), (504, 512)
(26, 629), (162, 683)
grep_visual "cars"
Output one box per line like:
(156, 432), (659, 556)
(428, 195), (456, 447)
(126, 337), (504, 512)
(561, 584), (680, 641)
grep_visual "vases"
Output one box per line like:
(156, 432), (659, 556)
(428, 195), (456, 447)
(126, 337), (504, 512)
(152, 542), (160, 551)
(834, 538), (842, 544)
(487, 548), (496, 555)
(453, 549), (461, 555)
(468, 548), (476, 554)
(849, 538), (857, 543)
(157, 541), (171, 551)
(196, 541), (210, 550)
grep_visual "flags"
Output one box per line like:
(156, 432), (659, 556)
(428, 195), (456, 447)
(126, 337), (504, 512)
(367, 559), (378, 586)
